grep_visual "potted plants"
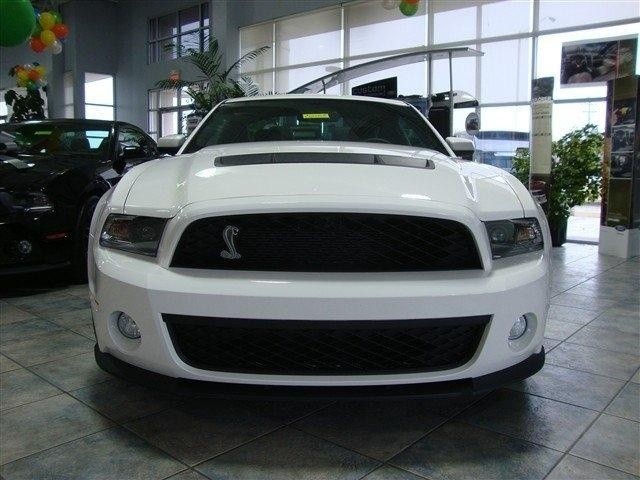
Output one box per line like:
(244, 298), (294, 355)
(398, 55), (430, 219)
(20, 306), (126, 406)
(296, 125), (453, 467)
(504, 123), (608, 247)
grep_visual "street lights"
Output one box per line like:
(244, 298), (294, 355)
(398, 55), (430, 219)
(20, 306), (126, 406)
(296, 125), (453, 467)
(513, 14), (558, 102)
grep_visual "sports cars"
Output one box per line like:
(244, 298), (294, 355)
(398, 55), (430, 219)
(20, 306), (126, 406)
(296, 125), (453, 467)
(86, 91), (555, 396)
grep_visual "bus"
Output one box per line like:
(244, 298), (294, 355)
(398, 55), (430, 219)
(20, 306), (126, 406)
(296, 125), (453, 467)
(452, 131), (530, 178)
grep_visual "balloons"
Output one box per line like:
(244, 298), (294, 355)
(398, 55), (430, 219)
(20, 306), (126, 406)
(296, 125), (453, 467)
(382, 0), (401, 10)
(30, 8), (70, 56)
(399, 1), (419, 17)
(14, 59), (49, 98)
(0, 0), (35, 48)
(403, 0), (421, 4)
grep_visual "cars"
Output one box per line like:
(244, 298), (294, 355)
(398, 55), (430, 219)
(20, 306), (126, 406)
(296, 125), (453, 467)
(0, 117), (165, 284)
